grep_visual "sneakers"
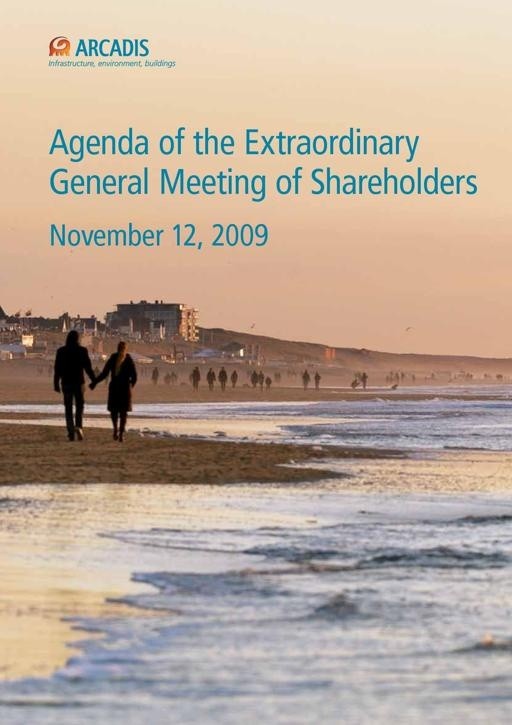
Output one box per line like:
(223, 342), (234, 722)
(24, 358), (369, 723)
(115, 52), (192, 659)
(75, 427), (83, 440)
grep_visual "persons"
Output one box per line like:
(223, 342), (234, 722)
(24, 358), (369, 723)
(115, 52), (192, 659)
(88, 340), (137, 442)
(206, 367), (216, 391)
(230, 369), (238, 388)
(246, 368), (503, 392)
(192, 366), (200, 392)
(37, 362), (192, 388)
(54, 330), (99, 442)
(218, 366), (227, 392)
